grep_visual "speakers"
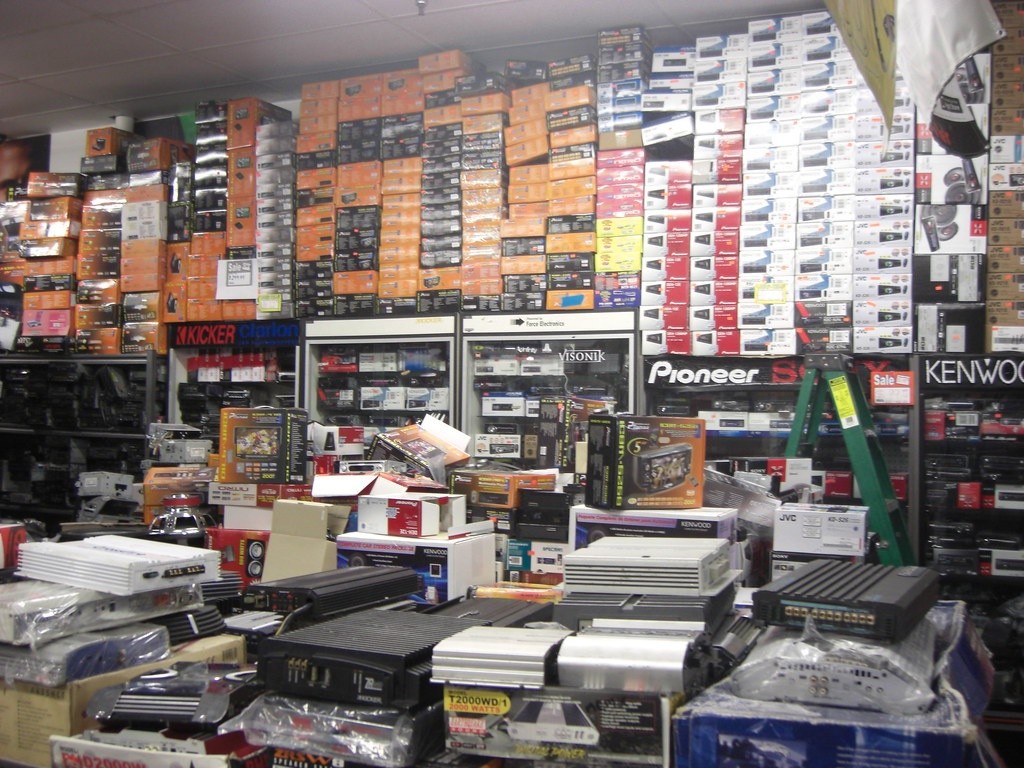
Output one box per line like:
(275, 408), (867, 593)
(245, 539), (265, 577)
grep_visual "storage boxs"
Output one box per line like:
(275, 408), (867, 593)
(444, 684), (714, 768)
(1, 1), (1024, 353)
(539, 398), (606, 474)
(705, 457), (910, 501)
(0, 633), (505, 767)
(870, 372), (913, 406)
(482, 391), (616, 417)
(318, 351), (442, 372)
(0, 518), (26, 569)
(361, 387), (450, 411)
(675, 599), (999, 767)
(474, 355), (566, 375)
(143, 408), (869, 605)
(926, 480), (982, 510)
(924, 411), (945, 441)
(188, 354), (266, 382)
(697, 410), (908, 438)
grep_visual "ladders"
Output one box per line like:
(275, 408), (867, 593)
(784, 353), (916, 566)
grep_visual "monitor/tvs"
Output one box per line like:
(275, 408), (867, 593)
(234, 425), (282, 459)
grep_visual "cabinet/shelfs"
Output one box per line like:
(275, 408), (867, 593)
(169, 318), (301, 453)
(460, 309), (641, 473)
(0, 350), (169, 514)
(302, 313), (460, 459)
(914, 352), (1024, 768)
(641, 354), (913, 566)
